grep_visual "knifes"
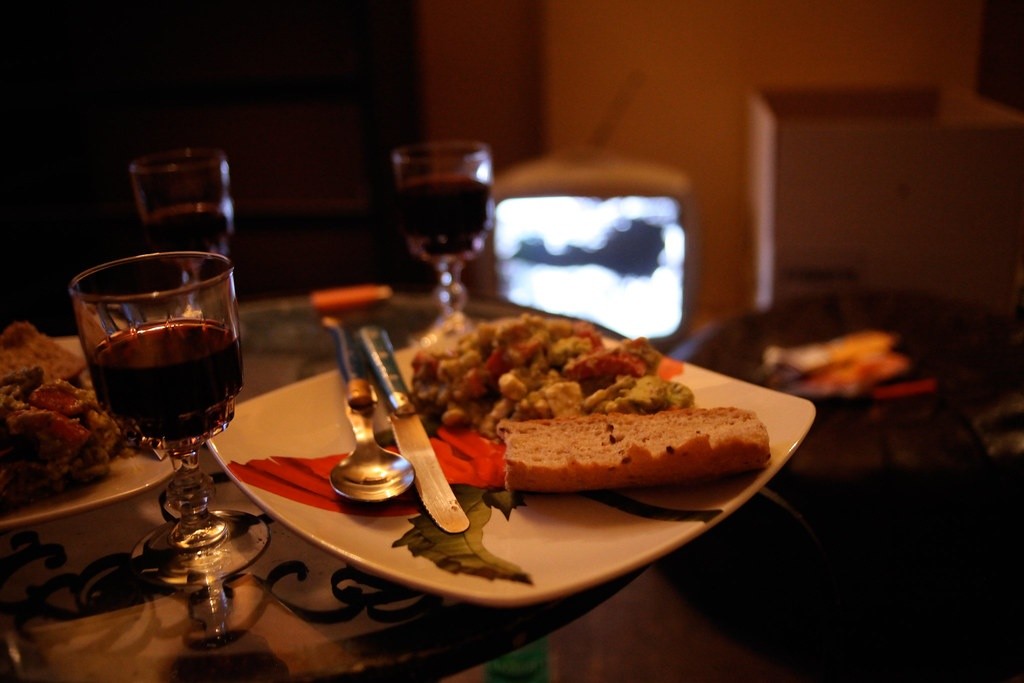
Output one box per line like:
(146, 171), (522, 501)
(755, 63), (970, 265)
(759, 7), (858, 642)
(359, 326), (470, 535)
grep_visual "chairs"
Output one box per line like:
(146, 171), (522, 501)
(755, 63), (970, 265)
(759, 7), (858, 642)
(0, 0), (435, 330)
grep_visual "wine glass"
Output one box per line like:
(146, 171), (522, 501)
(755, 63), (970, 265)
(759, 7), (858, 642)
(71, 247), (267, 586)
(396, 139), (497, 320)
(130, 146), (235, 318)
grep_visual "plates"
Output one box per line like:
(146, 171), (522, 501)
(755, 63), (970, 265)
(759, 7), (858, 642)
(207, 333), (815, 602)
(0, 454), (183, 527)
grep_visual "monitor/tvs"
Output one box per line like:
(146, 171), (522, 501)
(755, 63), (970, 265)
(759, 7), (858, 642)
(490, 160), (702, 353)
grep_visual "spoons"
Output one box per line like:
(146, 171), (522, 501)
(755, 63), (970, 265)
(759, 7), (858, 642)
(328, 327), (415, 501)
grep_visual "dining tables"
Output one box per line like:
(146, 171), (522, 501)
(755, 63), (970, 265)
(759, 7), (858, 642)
(1, 288), (798, 683)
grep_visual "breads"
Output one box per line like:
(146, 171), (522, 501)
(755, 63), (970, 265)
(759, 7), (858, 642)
(0, 320), (87, 387)
(498, 407), (769, 492)
